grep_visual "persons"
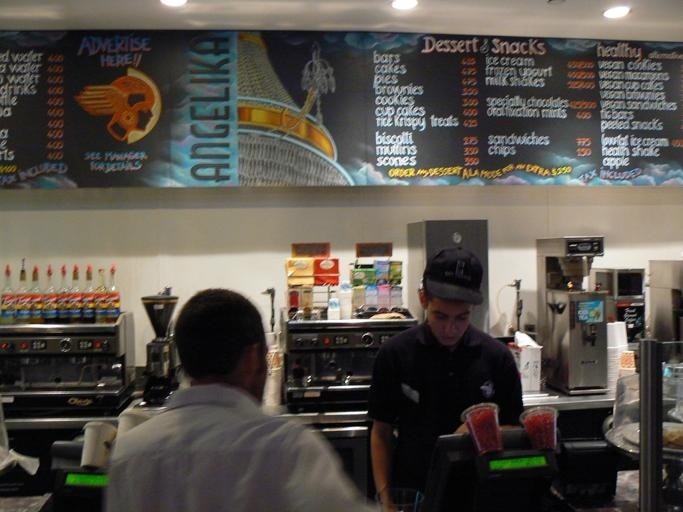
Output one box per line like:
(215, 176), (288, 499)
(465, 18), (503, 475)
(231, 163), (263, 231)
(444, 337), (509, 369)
(365, 244), (527, 512)
(100, 287), (389, 511)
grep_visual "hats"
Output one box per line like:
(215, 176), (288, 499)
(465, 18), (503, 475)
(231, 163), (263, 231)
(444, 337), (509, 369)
(423, 245), (485, 304)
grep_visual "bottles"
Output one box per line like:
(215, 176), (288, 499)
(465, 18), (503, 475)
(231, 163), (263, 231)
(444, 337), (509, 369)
(302, 285), (314, 310)
(353, 284), (402, 310)
(375, 260), (402, 284)
(327, 289), (340, 320)
(0, 256), (121, 325)
(288, 286), (299, 310)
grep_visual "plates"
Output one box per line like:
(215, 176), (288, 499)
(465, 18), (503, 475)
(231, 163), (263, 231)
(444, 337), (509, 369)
(622, 421), (683, 453)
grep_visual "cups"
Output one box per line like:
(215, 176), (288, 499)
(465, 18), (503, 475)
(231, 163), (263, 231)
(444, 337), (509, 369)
(519, 406), (560, 449)
(461, 403), (504, 455)
(115, 410), (152, 438)
(374, 487), (425, 512)
(263, 344), (282, 406)
(606, 321), (637, 392)
(80, 421), (117, 471)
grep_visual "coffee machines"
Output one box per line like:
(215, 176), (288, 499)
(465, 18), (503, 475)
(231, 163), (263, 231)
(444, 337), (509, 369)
(137, 286), (179, 408)
